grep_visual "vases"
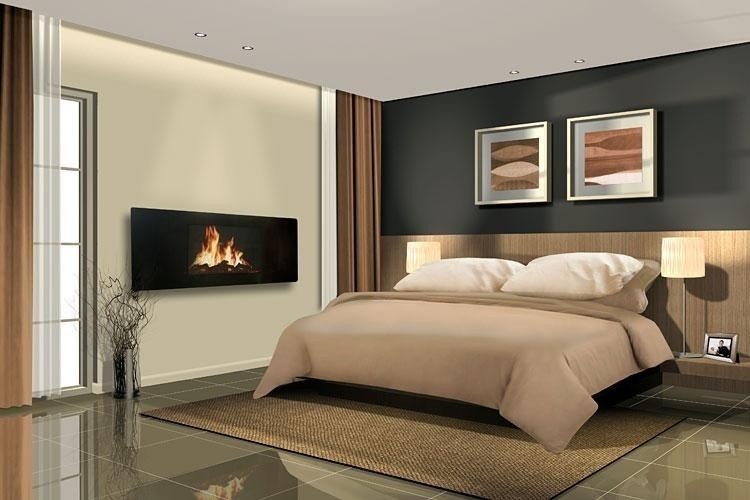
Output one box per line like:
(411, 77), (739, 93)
(111, 347), (142, 399)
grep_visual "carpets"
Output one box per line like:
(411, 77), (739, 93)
(139, 378), (690, 500)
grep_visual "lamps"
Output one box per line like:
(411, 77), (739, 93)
(509, 70), (519, 75)
(405, 241), (442, 274)
(242, 45), (253, 51)
(194, 32), (207, 38)
(660, 236), (706, 358)
(574, 59), (585, 64)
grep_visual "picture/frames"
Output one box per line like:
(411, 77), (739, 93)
(566, 107), (658, 202)
(702, 333), (739, 364)
(474, 120), (552, 205)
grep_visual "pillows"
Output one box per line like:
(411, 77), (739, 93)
(393, 257), (526, 293)
(587, 258), (660, 313)
(500, 251), (644, 300)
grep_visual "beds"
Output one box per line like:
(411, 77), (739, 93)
(253, 256), (675, 456)
